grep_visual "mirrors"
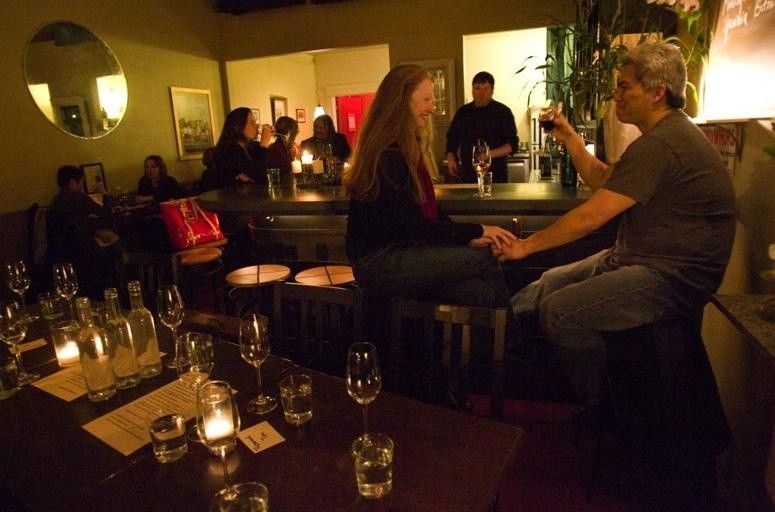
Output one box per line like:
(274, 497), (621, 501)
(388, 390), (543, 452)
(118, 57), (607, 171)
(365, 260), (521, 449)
(21, 19), (129, 141)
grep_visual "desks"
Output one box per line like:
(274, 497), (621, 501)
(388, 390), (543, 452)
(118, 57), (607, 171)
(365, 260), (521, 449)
(0, 293), (529, 512)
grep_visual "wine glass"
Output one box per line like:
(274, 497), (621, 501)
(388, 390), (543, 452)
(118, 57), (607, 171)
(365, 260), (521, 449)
(5, 260), (40, 326)
(51, 261), (79, 322)
(473, 142), (493, 195)
(158, 283), (217, 388)
(345, 338), (382, 431)
(237, 313), (279, 416)
(195, 380), (241, 480)
(0, 298), (41, 385)
(253, 121), (292, 142)
(321, 141), (336, 177)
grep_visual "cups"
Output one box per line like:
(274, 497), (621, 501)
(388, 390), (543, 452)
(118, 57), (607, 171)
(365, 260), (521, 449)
(349, 432), (397, 499)
(35, 291), (64, 321)
(480, 171), (493, 195)
(267, 167), (280, 188)
(0, 356), (21, 399)
(537, 98), (562, 132)
(50, 319), (80, 368)
(218, 479), (267, 511)
(278, 372), (313, 424)
(145, 405), (188, 466)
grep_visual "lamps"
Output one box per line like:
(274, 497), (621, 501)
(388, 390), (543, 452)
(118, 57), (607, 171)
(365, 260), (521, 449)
(311, 103), (327, 121)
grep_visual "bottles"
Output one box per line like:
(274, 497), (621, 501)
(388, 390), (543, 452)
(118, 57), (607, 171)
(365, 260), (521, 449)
(76, 280), (161, 406)
(561, 149), (579, 188)
(539, 139), (552, 177)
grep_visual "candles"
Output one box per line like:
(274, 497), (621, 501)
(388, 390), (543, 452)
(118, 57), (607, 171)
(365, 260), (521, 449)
(300, 154), (313, 165)
(311, 160), (324, 174)
(291, 160), (302, 174)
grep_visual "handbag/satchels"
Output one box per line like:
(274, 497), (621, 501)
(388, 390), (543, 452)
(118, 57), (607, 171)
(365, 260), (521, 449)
(157, 195), (226, 252)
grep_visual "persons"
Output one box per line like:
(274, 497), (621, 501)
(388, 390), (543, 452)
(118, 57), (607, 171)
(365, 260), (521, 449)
(343, 62), (535, 410)
(490, 45), (738, 418)
(131, 152), (178, 211)
(46, 164), (131, 312)
(201, 146), (217, 191)
(212, 104), (292, 305)
(443, 71), (520, 183)
(269, 116), (305, 174)
(300, 115), (352, 169)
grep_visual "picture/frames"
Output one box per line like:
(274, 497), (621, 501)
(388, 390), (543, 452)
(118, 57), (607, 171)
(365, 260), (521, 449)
(169, 85), (219, 162)
(295, 108), (307, 124)
(270, 96), (289, 127)
(249, 108), (261, 126)
(396, 56), (457, 130)
(79, 161), (108, 195)
